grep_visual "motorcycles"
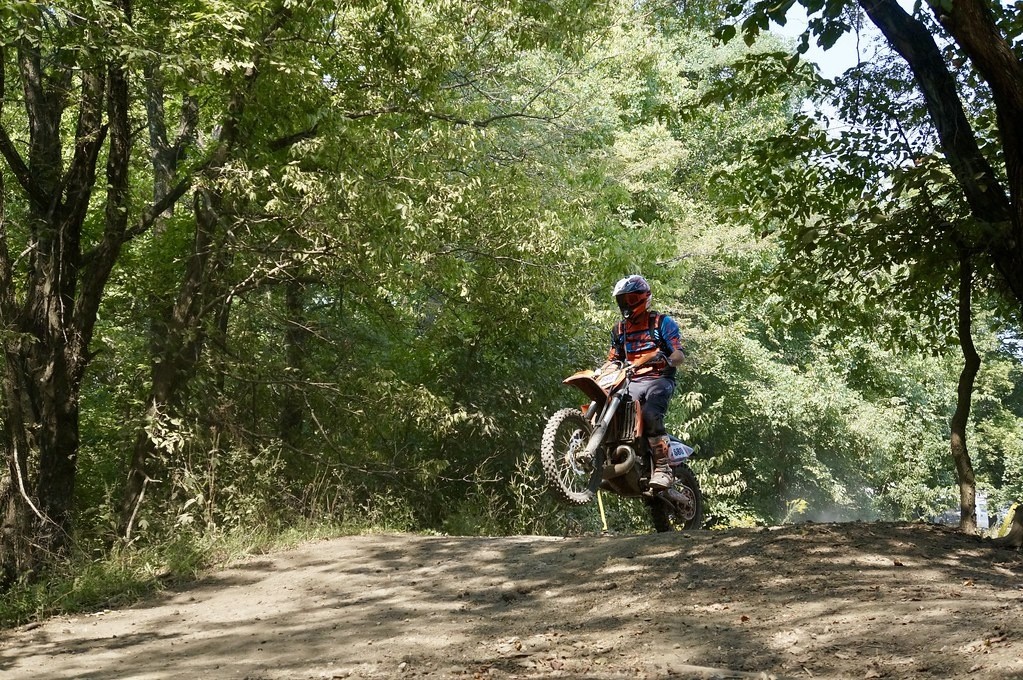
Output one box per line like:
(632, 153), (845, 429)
(540, 346), (703, 533)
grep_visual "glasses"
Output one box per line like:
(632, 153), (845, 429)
(616, 293), (640, 306)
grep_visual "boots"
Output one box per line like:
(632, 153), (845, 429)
(648, 433), (672, 489)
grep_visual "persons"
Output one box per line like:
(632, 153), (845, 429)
(594, 275), (685, 488)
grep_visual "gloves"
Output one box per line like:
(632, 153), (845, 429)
(653, 354), (672, 372)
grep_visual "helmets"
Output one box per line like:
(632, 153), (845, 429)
(612, 275), (652, 320)
